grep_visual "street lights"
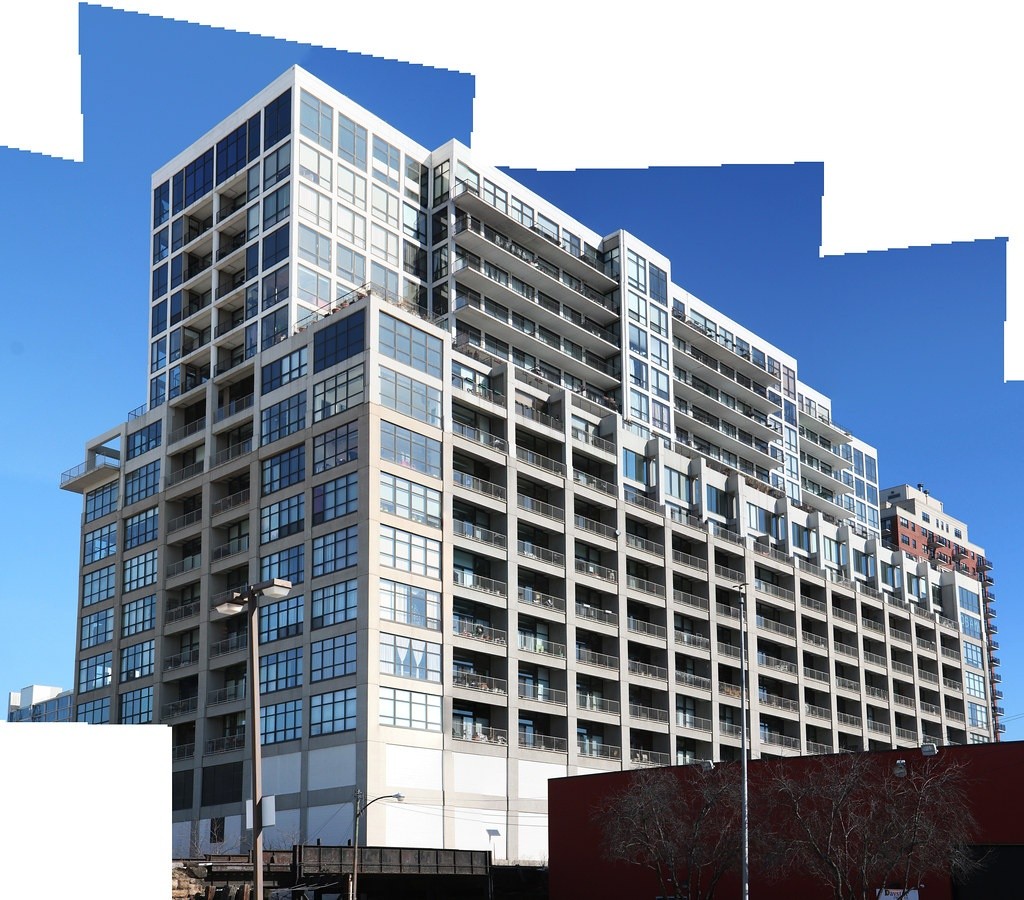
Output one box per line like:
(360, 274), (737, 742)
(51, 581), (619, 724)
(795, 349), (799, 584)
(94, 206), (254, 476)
(213, 578), (293, 900)
(353, 792), (406, 900)
